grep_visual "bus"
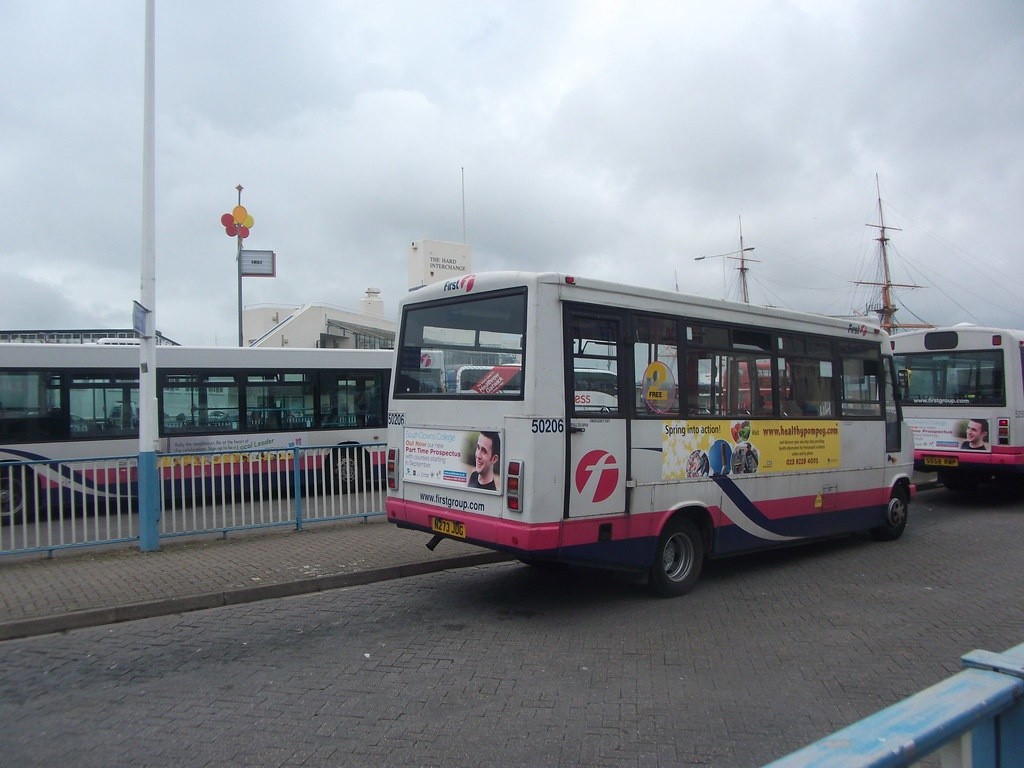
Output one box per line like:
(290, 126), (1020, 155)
(877, 323), (1024, 497)
(455, 363), (618, 412)
(0, 343), (446, 524)
(639, 372), (720, 411)
(386, 270), (916, 598)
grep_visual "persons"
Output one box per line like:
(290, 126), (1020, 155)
(465, 430), (500, 490)
(743, 444), (758, 473)
(958, 419), (989, 451)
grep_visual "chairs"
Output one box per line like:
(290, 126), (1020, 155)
(902, 394), (920, 401)
(922, 395), (935, 399)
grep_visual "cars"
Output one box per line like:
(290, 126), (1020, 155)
(70, 414), (98, 432)
(206, 410), (232, 427)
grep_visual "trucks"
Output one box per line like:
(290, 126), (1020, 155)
(722, 362), (790, 414)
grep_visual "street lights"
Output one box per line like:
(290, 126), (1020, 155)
(694, 248), (755, 298)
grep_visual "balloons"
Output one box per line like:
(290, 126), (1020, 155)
(221, 205), (254, 238)
